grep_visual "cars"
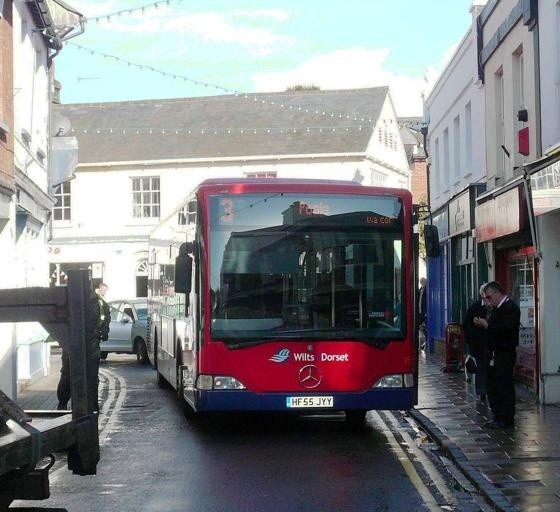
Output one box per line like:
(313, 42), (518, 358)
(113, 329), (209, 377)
(97, 300), (149, 364)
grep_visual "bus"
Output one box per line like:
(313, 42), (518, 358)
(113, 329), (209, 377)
(148, 177), (440, 430)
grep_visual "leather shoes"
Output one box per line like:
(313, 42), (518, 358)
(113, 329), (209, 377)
(485, 421), (515, 430)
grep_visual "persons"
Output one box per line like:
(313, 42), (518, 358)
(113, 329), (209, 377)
(98, 283), (108, 304)
(417, 277), (426, 327)
(473, 282), (520, 431)
(465, 283), (494, 405)
(57, 281), (112, 412)
